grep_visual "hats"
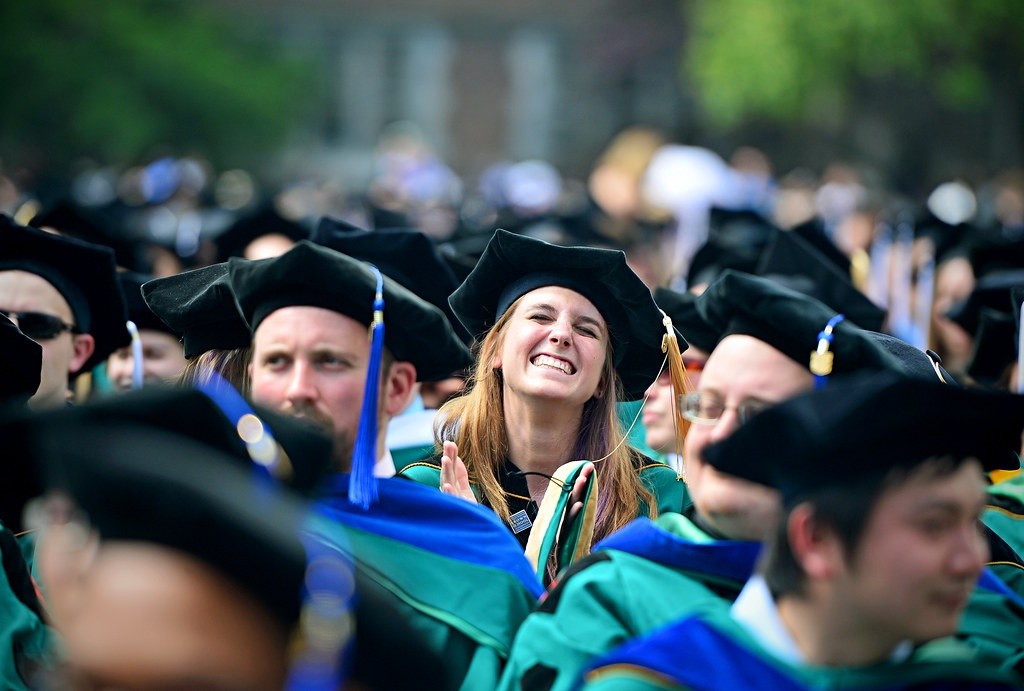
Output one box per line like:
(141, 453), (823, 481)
(695, 367), (1024, 509)
(1, 156), (1024, 513)
(0, 381), (356, 691)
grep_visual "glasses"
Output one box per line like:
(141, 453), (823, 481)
(680, 391), (781, 428)
(0, 310), (83, 340)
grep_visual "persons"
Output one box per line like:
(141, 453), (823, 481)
(226, 241), (543, 603)
(399, 229), (693, 596)
(0, 387), (523, 691)
(575, 370), (1024, 691)
(0, 200), (480, 469)
(638, 202), (1024, 558)
(494, 270), (1024, 691)
